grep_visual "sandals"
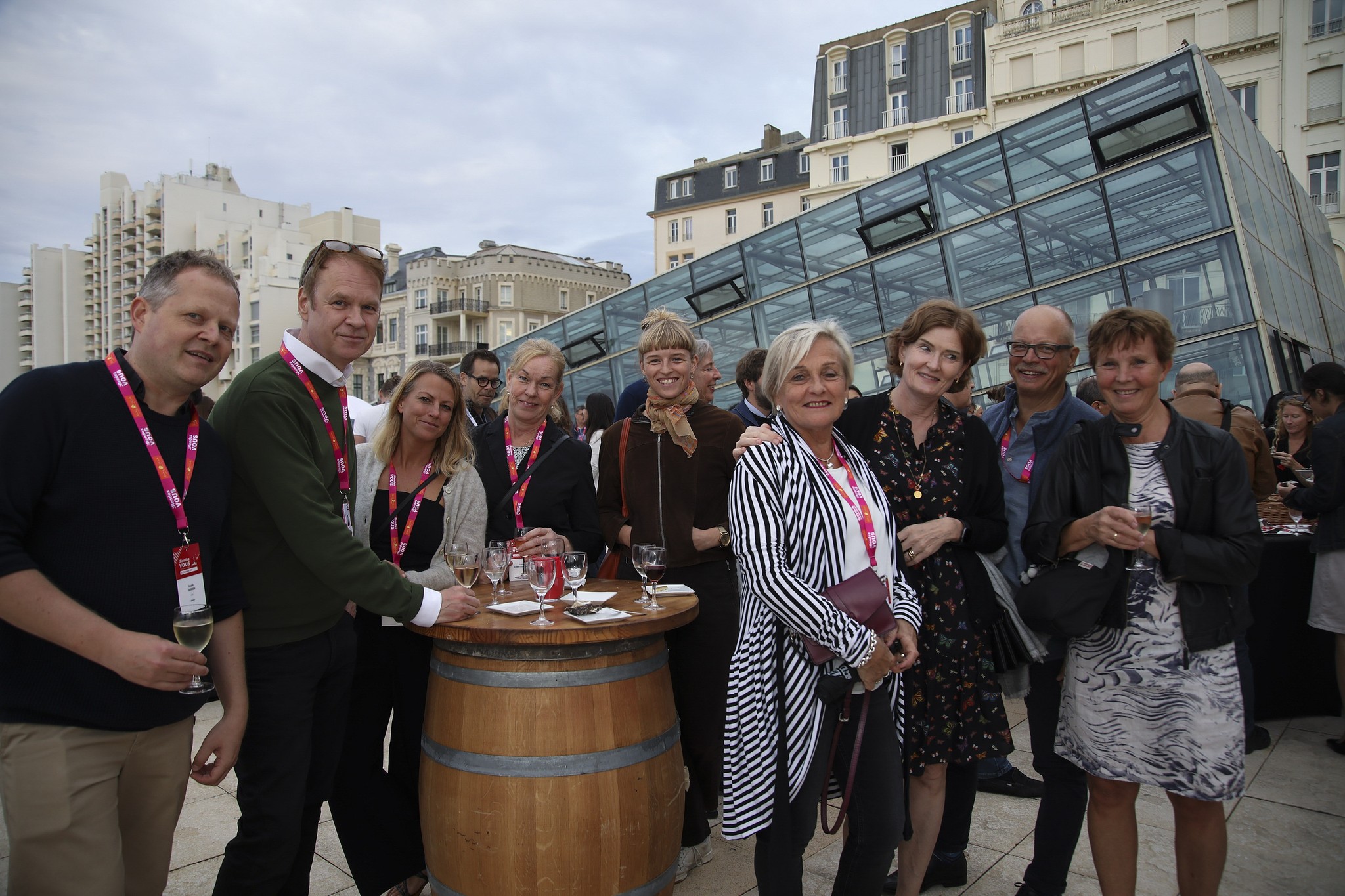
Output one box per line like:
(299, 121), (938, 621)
(386, 872), (429, 896)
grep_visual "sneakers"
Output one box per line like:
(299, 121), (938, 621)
(675, 834), (712, 885)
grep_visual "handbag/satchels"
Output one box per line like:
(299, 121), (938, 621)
(797, 566), (897, 667)
(596, 549), (621, 579)
(1015, 539), (1127, 639)
(953, 542), (1020, 630)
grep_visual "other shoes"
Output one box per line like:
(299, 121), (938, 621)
(1244, 725), (1272, 750)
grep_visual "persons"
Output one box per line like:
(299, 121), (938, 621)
(1, 238), (1344, 896)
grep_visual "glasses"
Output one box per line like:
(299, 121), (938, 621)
(462, 371), (503, 389)
(1006, 341), (1075, 359)
(1300, 390), (1317, 410)
(301, 240), (384, 287)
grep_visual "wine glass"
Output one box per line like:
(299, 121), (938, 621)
(527, 557), (555, 626)
(513, 526), (535, 580)
(481, 547), (508, 607)
(444, 541), (468, 586)
(1121, 502), (1153, 571)
(1288, 508), (1303, 535)
(173, 604), (215, 694)
(489, 539), (514, 596)
(453, 552), (481, 616)
(632, 543), (656, 603)
(641, 548), (668, 611)
(541, 538), (565, 560)
(561, 551), (588, 608)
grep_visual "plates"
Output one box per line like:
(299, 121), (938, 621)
(486, 600), (554, 617)
(1306, 478), (1314, 482)
(563, 607), (632, 624)
(564, 579), (585, 589)
(1295, 470), (1313, 472)
(559, 591), (617, 606)
(640, 584), (695, 597)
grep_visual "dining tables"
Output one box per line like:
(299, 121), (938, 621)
(402, 578), (700, 896)
(1248, 523), (1342, 722)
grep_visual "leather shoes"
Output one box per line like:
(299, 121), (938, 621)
(1014, 882), (1062, 896)
(881, 850), (968, 896)
(976, 767), (1045, 798)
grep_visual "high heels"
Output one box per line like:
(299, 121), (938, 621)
(1332, 740), (1345, 754)
(1326, 739), (1339, 744)
